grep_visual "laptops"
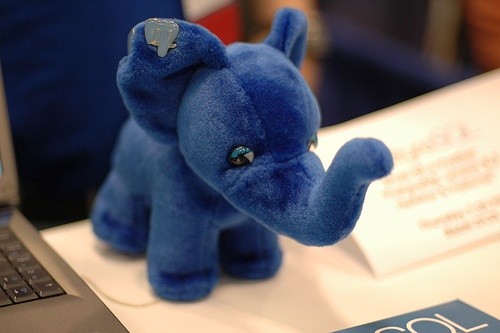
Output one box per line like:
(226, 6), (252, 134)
(1, 71), (129, 333)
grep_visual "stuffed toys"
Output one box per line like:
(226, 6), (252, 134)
(90, 9), (393, 302)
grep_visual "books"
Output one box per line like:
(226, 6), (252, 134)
(331, 299), (500, 333)
